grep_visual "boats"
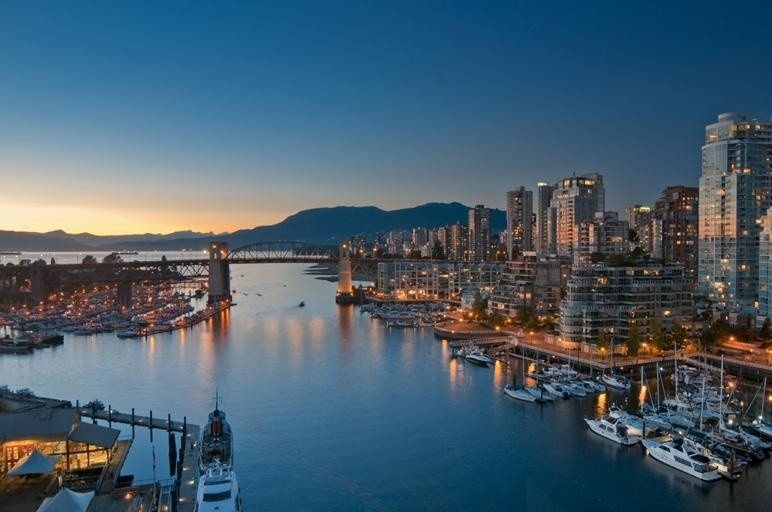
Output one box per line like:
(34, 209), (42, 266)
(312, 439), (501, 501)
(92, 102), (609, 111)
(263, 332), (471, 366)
(193, 408), (241, 512)
(453, 341), (493, 365)
(0, 336), (34, 353)
(682, 433), (744, 480)
(641, 431), (722, 482)
(1, 251), (23, 256)
(359, 300), (449, 327)
(110, 249), (139, 255)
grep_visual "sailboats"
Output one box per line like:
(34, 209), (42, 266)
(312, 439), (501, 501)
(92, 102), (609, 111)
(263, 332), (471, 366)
(525, 347), (608, 403)
(583, 340), (772, 465)
(595, 336), (631, 390)
(503, 344), (537, 402)
